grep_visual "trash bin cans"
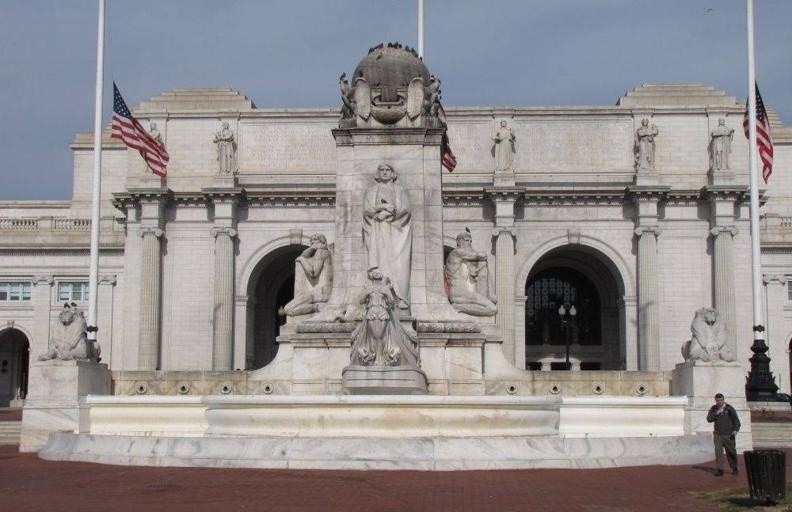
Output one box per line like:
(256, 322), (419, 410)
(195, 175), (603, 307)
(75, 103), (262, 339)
(743, 449), (786, 506)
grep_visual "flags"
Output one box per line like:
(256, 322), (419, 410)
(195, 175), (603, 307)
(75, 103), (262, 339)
(743, 80), (773, 184)
(437, 104), (457, 172)
(110, 81), (169, 176)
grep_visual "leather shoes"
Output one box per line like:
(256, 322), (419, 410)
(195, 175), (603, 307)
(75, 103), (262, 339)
(734, 469), (738, 475)
(715, 470), (722, 476)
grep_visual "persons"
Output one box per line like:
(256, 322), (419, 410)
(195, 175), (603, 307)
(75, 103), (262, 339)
(146, 122), (161, 173)
(446, 231), (497, 316)
(711, 117), (735, 170)
(637, 118), (658, 171)
(707, 394), (741, 476)
(351, 265), (418, 366)
(495, 120), (513, 171)
(218, 121), (234, 173)
(284, 233), (333, 316)
(362, 162), (412, 300)
